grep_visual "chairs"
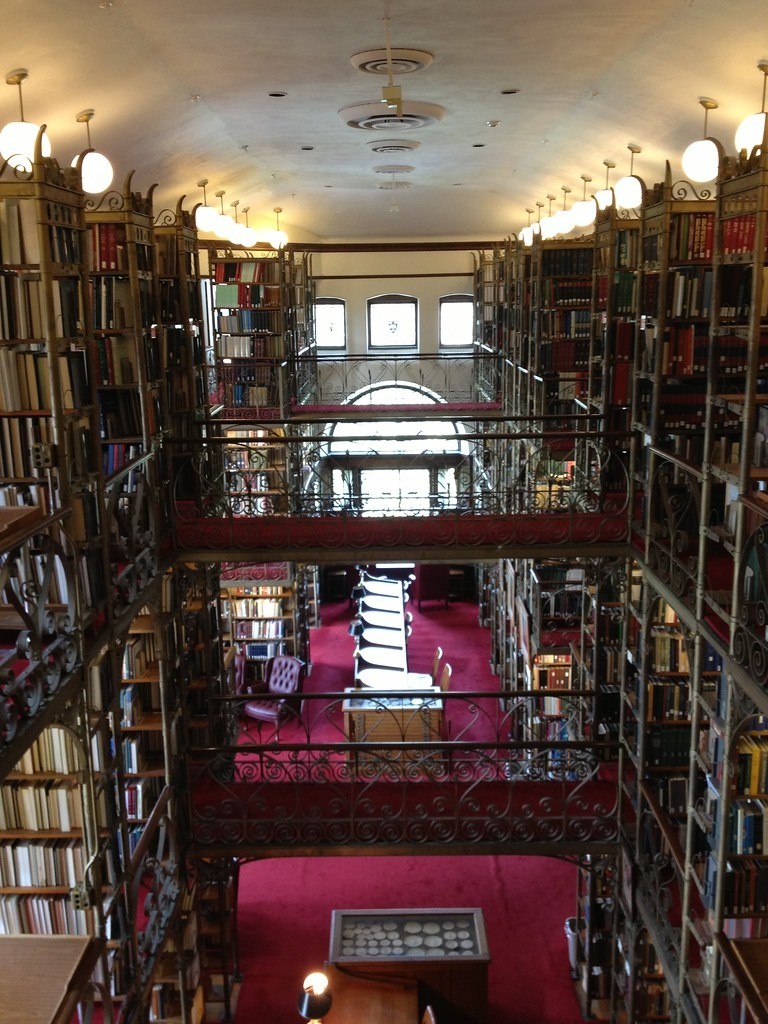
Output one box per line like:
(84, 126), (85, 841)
(245, 655), (307, 741)
(405, 611), (414, 625)
(422, 1005), (438, 1024)
(403, 592), (410, 606)
(403, 580), (409, 592)
(440, 663), (453, 702)
(432, 646), (443, 683)
(405, 624), (413, 644)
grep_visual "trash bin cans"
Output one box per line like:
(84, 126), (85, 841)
(564, 917), (587, 969)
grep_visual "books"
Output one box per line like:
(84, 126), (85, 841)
(0, 193), (322, 1024)
(472, 210), (768, 1024)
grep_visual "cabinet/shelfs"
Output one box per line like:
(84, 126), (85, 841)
(471, 113), (768, 1024)
(327, 569), (347, 602)
(446, 569), (465, 602)
(0, 123), (323, 1024)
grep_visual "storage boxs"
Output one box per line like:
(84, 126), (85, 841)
(565, 916), (577, 970)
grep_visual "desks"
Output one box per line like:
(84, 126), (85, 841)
(355, 634), (407, 670)
(342, 686), (444, 776)
(354, 657), (434, 687)
(361, 571), (404, 596)
(359, 617), (405, 647)
(320, 962), (420, 1024)
(358, 601), (404, 629)
(359, 586), (404, 613)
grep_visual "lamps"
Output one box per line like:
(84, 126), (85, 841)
(216, 191), (235, 239)
(347, 620), (365, 643)
(518, 208), (533, 247)
(268, 206), (288, 250)
(735, 59), (768, 160)
(681, 97), (719, 182)
(296, 972), (333, 1024)
(0, 68), (51, 172)
(614, 143), (642, 209)
(241, 206), (258, 248)
(531, 202), (547, 241)
(197, 179), (221, 232)
(71, 109), (113, 195)
(540, 194), (558, 237)
(553, 185), (575, 235)
(572, 174), (596, 227)
(354, 564), (369, 576)
(594, 159), (619, 210)
(230, 200), (247, 245)
(351, 586), (367, 605)
